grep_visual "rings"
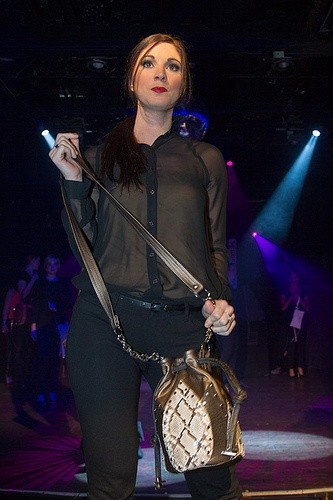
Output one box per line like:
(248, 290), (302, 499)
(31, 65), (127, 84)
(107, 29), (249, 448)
(54, 144), (58, 149)
(224, 311), (231, 321)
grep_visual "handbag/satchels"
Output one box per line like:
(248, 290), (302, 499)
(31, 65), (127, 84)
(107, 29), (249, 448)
(290, 309), (304, 330)
(152, 349), (247, 474)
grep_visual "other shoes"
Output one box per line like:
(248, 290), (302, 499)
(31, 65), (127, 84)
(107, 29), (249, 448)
(289, 376), (296, 379)
(300, 375), (305, 378)
(6, 383), (13, 388)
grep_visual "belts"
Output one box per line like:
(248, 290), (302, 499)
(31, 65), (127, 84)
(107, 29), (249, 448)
(81, 288), (202, 312)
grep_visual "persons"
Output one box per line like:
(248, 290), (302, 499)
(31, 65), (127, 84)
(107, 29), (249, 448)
(2, 249), (76, 415)
(49, 34), (243, 500)
(225, 264), (310, 381)
